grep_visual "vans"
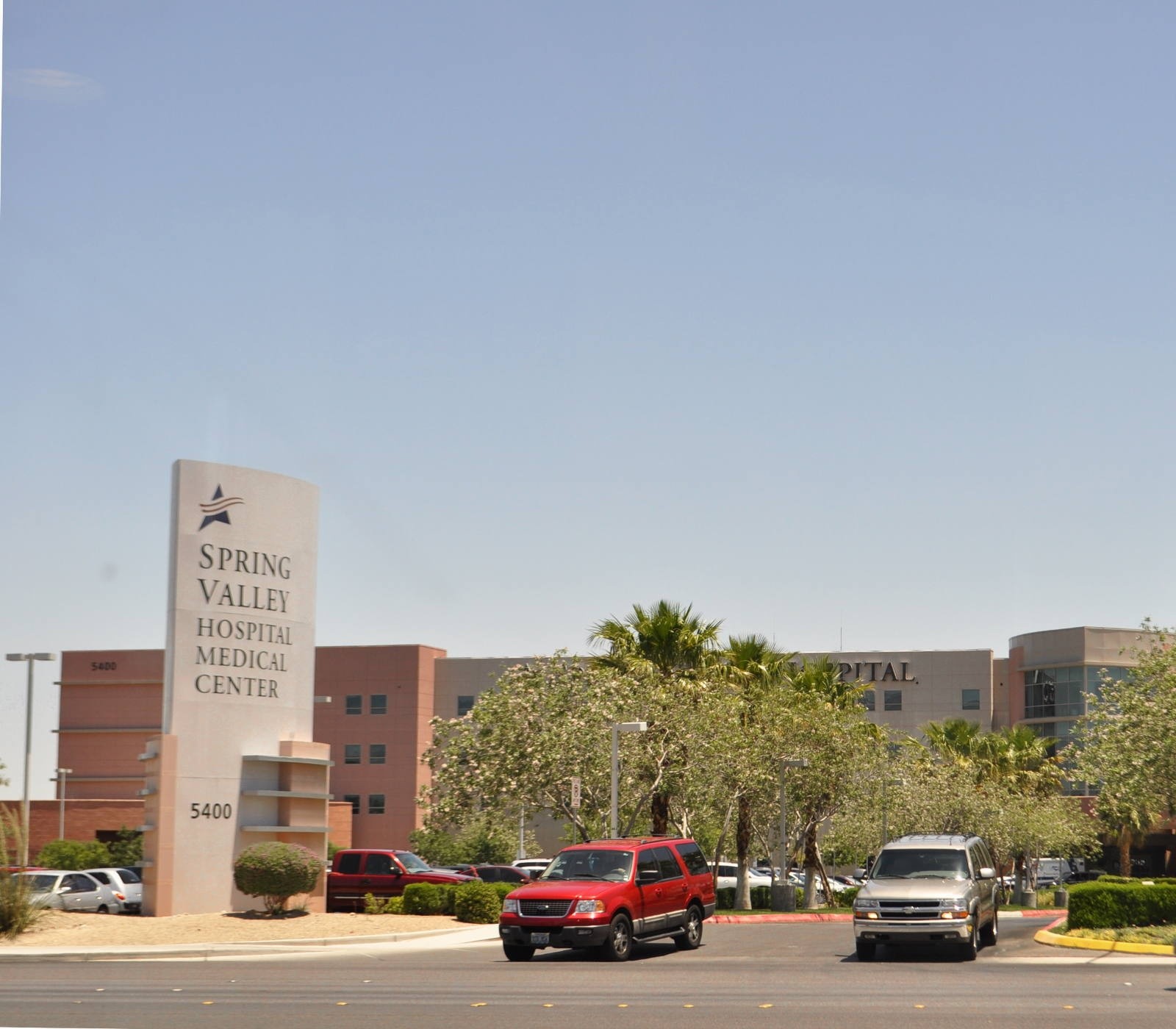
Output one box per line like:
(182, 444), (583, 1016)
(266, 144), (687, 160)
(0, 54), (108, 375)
(1030, 858), (1071, 879)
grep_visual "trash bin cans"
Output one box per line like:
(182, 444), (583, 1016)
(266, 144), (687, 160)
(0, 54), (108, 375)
(1055, 892), (1066, 907)
(771, 886), (795, 912)
(528, 870), (544, 879)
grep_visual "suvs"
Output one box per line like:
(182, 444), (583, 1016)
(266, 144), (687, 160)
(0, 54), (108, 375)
(1040, 871), (1107, 889)
(853, 832), (998, 961)
(499, 834), (716, 962)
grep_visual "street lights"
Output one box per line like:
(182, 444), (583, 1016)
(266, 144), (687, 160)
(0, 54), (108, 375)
(882, 779), (903, 847)
(55, 768), (73, 840)
(6, 653), (58, 864)
(612, 721), (647, 838)
(779, 757), (807, 879)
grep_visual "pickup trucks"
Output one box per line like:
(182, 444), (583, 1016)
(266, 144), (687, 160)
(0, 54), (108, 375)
(327, 849), (483, 912)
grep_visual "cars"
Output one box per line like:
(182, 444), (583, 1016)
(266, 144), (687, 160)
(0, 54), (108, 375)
(698, 861), (866, 906)
(429, 859), (582, 884)
(1, 865), (142, 914)
(996, 875), (1057, 891)
